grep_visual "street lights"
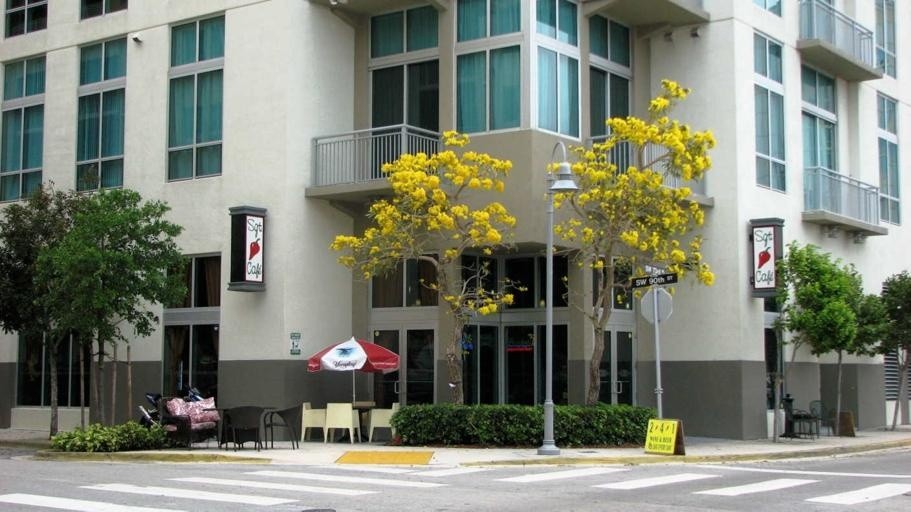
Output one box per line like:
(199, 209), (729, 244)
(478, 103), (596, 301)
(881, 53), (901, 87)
(537, 139), (578, 456)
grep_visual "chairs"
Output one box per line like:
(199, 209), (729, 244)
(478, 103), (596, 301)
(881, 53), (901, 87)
(786, 400), (836, 441)
(159, 394), (303, 452)
(301, 401), (401, 444)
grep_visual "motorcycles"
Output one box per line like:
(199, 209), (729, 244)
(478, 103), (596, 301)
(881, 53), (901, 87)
(137, 384), (205, 431)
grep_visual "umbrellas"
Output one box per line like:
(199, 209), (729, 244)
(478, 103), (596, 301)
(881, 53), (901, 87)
(306, 336), (400, 409)
